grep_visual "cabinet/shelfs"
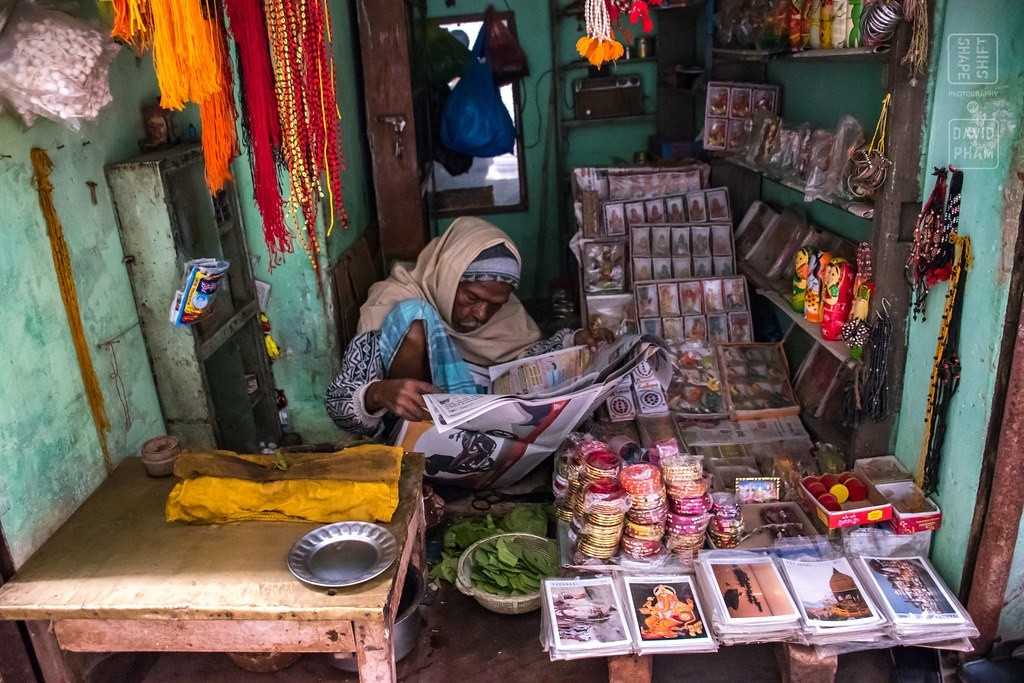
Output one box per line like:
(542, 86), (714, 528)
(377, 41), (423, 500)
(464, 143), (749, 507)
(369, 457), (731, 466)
(702, 1), (937, 467)
(548, 0), (696, 278)
(355, 0), (439, 277)
(103, 139), (284, 452)
(648, 0), (704, 163)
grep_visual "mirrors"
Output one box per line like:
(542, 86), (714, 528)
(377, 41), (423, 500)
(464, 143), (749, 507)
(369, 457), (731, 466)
(424, 9), (528, 219)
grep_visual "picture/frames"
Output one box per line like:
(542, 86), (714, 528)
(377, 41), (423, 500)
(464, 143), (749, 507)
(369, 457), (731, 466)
(735, 477), (781, 503)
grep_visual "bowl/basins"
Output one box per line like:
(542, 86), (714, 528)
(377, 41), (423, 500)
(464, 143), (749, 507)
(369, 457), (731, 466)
(456, 532), (560, 615)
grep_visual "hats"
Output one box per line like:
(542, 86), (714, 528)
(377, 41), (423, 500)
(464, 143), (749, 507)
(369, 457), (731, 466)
(461, 243), (520, 291)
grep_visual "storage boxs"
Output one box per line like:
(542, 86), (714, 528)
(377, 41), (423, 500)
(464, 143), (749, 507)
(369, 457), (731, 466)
(703, 80), (779, 152)
(854, 454), (915, 487)
(571, 167), (845, 422)
(706, 501), (821, 549)
(798, 470), (891, 529)
(875, 482), (942, 536)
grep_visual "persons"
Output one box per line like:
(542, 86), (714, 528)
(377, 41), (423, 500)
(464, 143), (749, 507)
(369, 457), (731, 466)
(541, 362), (557, 372)
(746, 482), (777, 500)
(324, 215), (616, 442)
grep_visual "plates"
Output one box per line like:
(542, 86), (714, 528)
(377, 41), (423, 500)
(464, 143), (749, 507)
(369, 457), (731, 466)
(287, 520), (398, 586)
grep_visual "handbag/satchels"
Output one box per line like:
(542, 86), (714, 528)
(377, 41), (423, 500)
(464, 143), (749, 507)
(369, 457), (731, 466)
(416, 15), (470, 88)
(484, 8), (530, 89)
(426, 104), (474, 176)
(440, 27), (516, 157)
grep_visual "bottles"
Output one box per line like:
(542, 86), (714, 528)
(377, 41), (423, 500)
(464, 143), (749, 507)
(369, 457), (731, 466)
(143, 102), (180, 146)
(424, 485), (447, 532)
(141, 434), (181, 476)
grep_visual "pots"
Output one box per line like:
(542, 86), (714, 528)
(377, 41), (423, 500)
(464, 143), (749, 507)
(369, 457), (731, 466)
(325, 565), (426, 671)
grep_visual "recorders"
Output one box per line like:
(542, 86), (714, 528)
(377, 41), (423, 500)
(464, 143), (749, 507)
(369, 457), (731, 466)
(573, 73), (645, 120)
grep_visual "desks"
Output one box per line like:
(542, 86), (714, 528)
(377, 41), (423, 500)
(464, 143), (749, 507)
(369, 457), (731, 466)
(0, 451), (429, 683)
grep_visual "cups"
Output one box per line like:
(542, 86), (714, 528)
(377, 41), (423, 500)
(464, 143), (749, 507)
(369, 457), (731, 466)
(622, 46), (633, 59)
(634, 35), (651, 59)
